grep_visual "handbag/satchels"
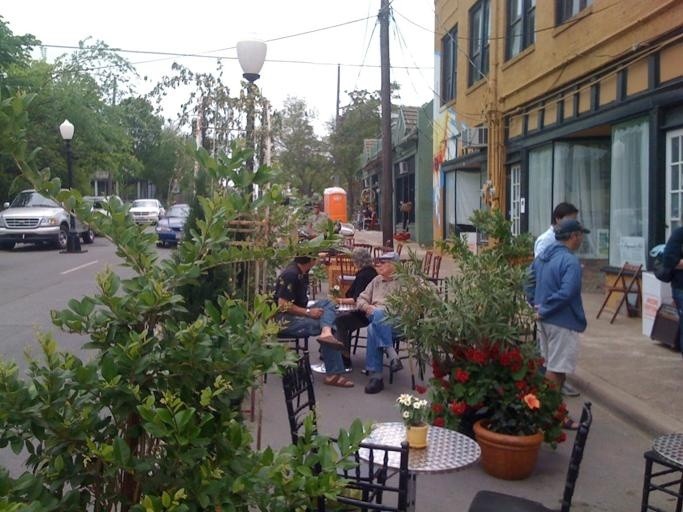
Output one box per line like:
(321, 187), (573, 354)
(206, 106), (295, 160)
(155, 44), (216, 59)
(653, 244), (673, 282)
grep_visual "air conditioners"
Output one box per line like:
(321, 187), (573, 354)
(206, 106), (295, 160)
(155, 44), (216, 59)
(461, 128), (487, 149)
(399, 162), (409, 174)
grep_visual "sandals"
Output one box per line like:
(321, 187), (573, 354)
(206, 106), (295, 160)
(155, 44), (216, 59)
(563, 418), (578, 430)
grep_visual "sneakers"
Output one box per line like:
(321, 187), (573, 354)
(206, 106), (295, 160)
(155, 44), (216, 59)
(311, 362), (327, 373)
(562, 382), (580, 397)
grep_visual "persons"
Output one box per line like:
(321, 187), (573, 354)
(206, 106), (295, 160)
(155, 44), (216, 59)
(660, 226), (683, 359)
(269, 200), (420, 395)
(527, 203), (591, 432)
(533, 203), (583, 400)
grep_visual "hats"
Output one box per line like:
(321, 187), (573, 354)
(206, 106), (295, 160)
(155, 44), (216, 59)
(555, 218), (591, 234)
(378, 252), (401, 262)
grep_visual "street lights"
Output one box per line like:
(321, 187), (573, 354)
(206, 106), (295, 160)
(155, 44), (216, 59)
(60, 117), (83, 254)
(233, 35), (269, 242)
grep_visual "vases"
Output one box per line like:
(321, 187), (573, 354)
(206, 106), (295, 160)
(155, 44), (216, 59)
(472, 419), (544, 479)
(405, 421), (428, 448)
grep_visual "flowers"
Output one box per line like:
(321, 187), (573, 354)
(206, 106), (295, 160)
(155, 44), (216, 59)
(395, 393), (429, 430)
(415, 334), (568, 450)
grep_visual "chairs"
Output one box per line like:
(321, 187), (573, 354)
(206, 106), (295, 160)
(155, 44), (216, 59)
(468, 401), (592, 512)
(641, 450), (683, 512)
(597, 262), (643, 323)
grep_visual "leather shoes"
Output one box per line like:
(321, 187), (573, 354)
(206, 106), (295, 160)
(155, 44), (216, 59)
(364, 377), (383, 393)
(389, 359), (403, 372)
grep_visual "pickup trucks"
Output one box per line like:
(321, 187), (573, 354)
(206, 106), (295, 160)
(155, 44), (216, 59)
(0, 188), (95, 249)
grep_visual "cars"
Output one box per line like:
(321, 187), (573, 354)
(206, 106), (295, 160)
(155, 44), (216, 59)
(127, 196), (164, 226)
(155, 201), (191, 250)
(82, 192), (123, 233)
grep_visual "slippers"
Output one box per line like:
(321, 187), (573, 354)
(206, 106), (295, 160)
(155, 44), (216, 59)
(317, 335), (345, 350)
(323, 375), (354, 387)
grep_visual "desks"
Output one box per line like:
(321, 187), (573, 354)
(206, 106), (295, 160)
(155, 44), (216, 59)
(652, 433), (683, 469)
(351, 422), (482, 512)
